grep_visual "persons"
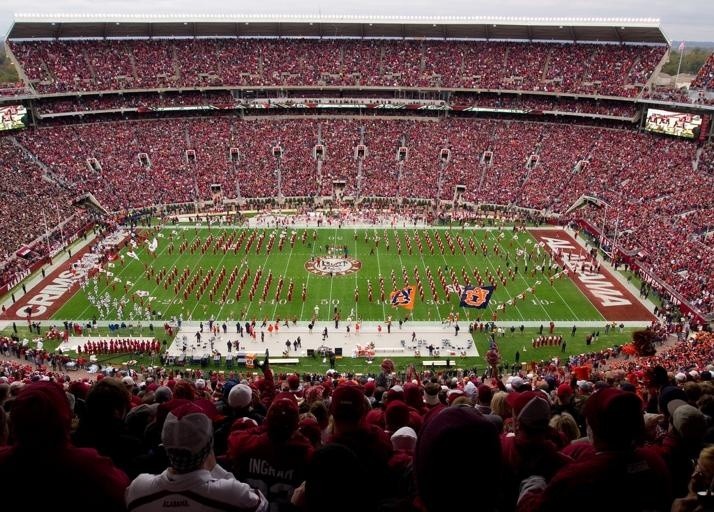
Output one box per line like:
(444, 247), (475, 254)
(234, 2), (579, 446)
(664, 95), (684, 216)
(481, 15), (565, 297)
(1, 38), (714, 511)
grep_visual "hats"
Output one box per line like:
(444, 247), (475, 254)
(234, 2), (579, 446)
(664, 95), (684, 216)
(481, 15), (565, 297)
(418, 365), (706, 476)
(9, 378), (214, 461)
(224, 373), (420, 442)
